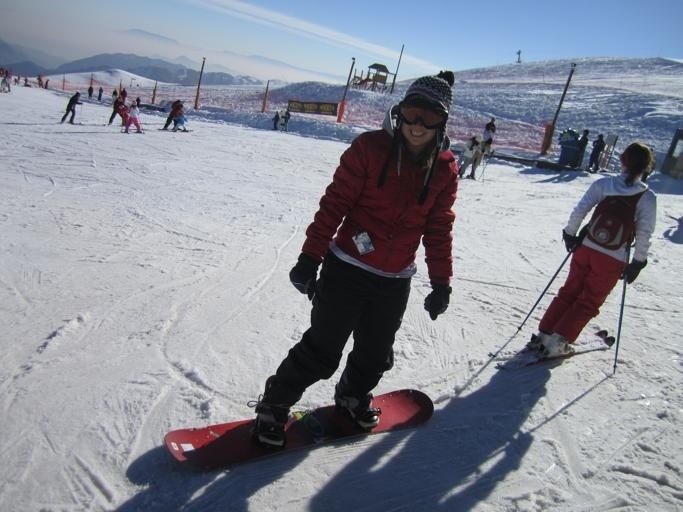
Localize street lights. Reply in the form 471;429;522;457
541;61;579;155
193;57;205;110
335;56;356;125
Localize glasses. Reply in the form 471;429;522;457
398;102;448;130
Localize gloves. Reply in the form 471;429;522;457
621;257;648;284
563;229;577;251
424;284;452;320
289;254;320;300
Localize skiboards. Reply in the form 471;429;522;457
490;330;614;369
121;130;146;135
157;128;193;132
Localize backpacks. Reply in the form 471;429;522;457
587;190;648;250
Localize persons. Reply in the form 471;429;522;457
256;70;457;450
88;86;188;133
281;109;291;131
528;143;656;360
455;117;495;179
0;68;49;92
589;133;605;171
572;130;588;168
61;92;82;124
272;111;279;129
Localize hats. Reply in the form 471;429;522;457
405;70;455;120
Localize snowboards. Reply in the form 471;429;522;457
165;390;434;471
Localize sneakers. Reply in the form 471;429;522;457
255;400;290;447
334;385;379;428
536;332;575;357
